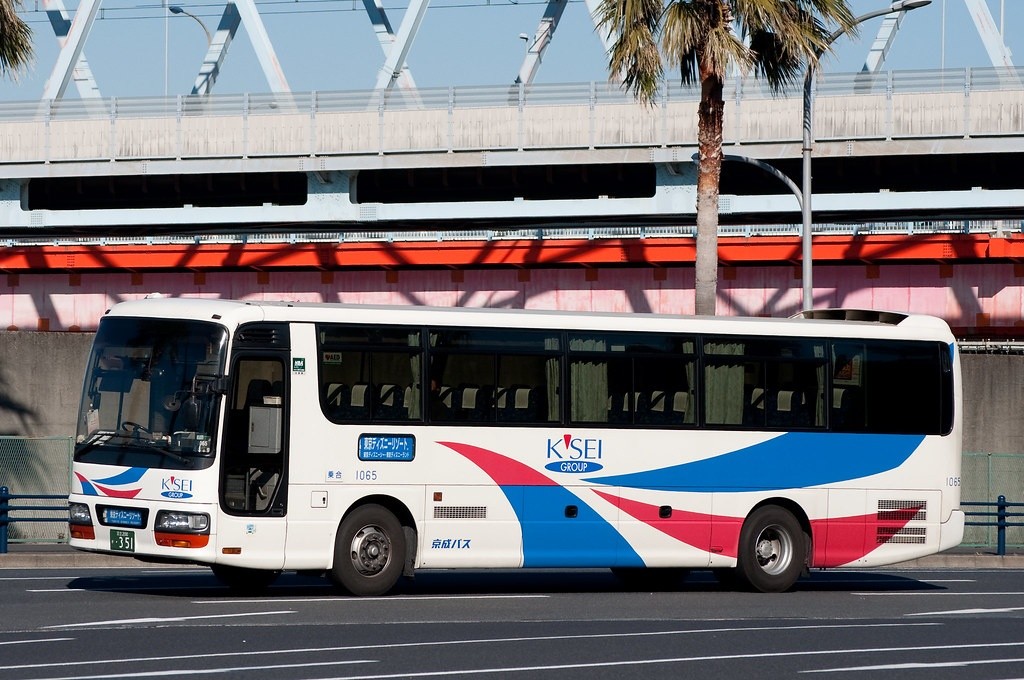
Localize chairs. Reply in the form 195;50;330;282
747;388;806;428
236;379;282;446
438;386;543;422
320;382;417;424
607;392;687;425
834;388;863;428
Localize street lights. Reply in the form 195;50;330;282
693;0;930;313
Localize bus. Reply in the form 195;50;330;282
65;298;966;595
